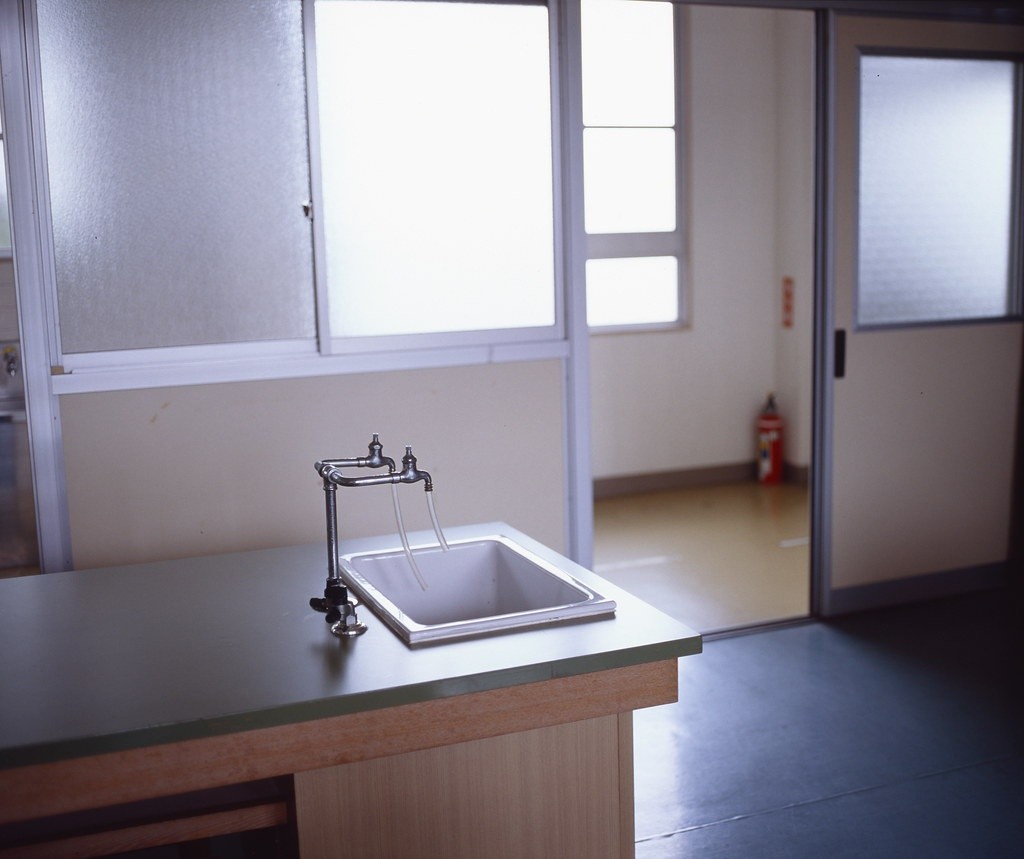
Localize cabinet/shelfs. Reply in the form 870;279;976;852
0;657;680;859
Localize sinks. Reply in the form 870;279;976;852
336;533;616;651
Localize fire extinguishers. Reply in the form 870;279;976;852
756;394;786;484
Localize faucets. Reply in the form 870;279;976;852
390;445;433;492
356;433;396;473
